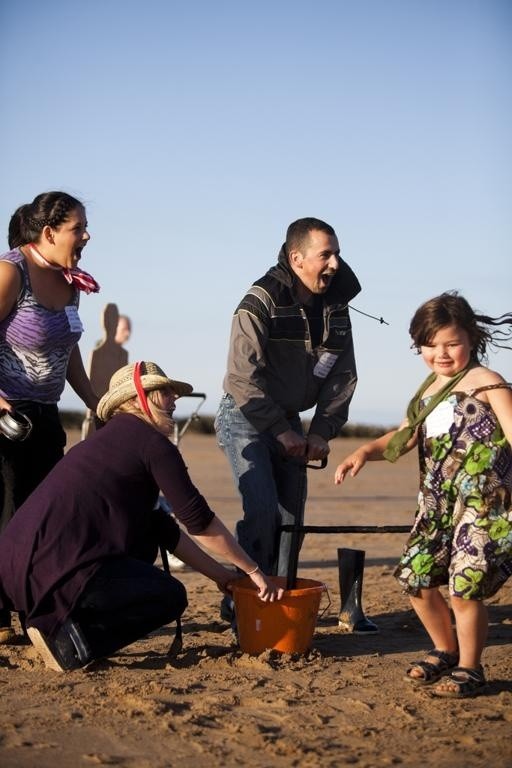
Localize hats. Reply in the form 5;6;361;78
96;361;195;422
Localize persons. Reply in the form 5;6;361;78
0;190;103;530
331;288;512;701
3;359;286;674
83;302;134;441
210;214;363;643
82;315;131;439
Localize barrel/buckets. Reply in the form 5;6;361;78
226;575;333;655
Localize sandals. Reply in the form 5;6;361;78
422;661;493;705
402;647;457;685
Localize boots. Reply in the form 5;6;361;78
333;544;385;640
219;516;291;631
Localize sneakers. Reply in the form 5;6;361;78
21;613;82;677
